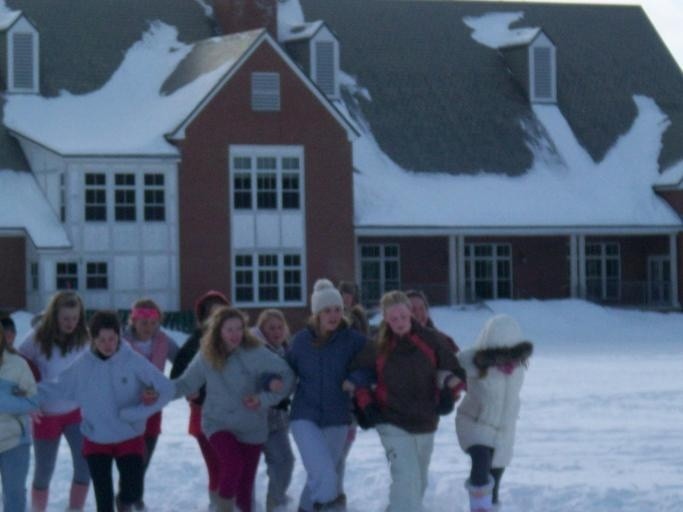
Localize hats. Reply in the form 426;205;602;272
88;309;119;339
311;279;344;313
194;291;230;315
131;300;161;320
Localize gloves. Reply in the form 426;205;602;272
446;377;467;395
242;395;261;410
138;389;160;406
358;390;381;423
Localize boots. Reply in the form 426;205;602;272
216;495;237;511
208;489;218;509
32;486;50;510
464;474;495;512
69;483;89;510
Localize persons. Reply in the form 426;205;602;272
170;290;233;511
253;309;295;511
406;289;459;360
11;309;175;512
435;315;534;512
0;324;36;512
260;278;377;512
120;299;200;511
16;288;95;512
337;279;370;483
142;305;296;512
352;289;466;512
3;319;42;396
